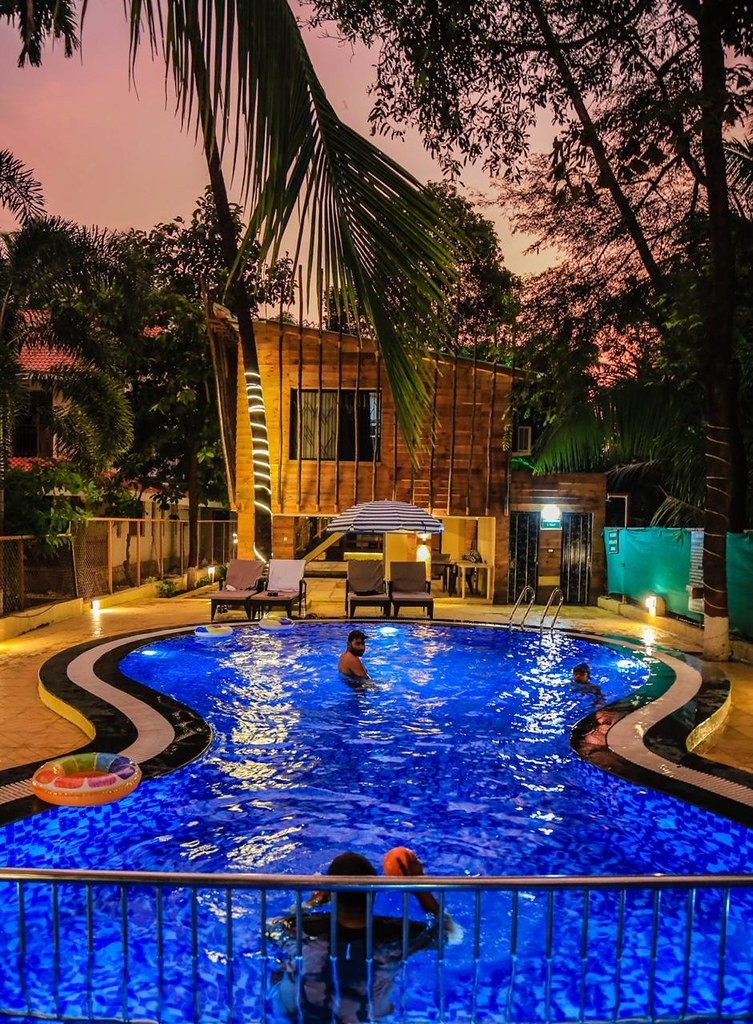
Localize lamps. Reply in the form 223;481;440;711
645;595;667;616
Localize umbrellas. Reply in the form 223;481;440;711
325;497;444;578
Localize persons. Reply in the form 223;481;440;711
338;630;370;679
572;664;591;683
294;851;443;939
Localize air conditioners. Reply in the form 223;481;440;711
517;425;532;453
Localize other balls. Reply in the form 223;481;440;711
384;844;418;880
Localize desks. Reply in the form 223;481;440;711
451;559;491;599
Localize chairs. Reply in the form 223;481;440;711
345;559;434;619
210;559;307;620
431;554;450;592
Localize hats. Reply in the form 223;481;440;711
348;631;370;642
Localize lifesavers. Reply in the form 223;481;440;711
30;751;144;811
194;622;233;639
258;616;297;632
